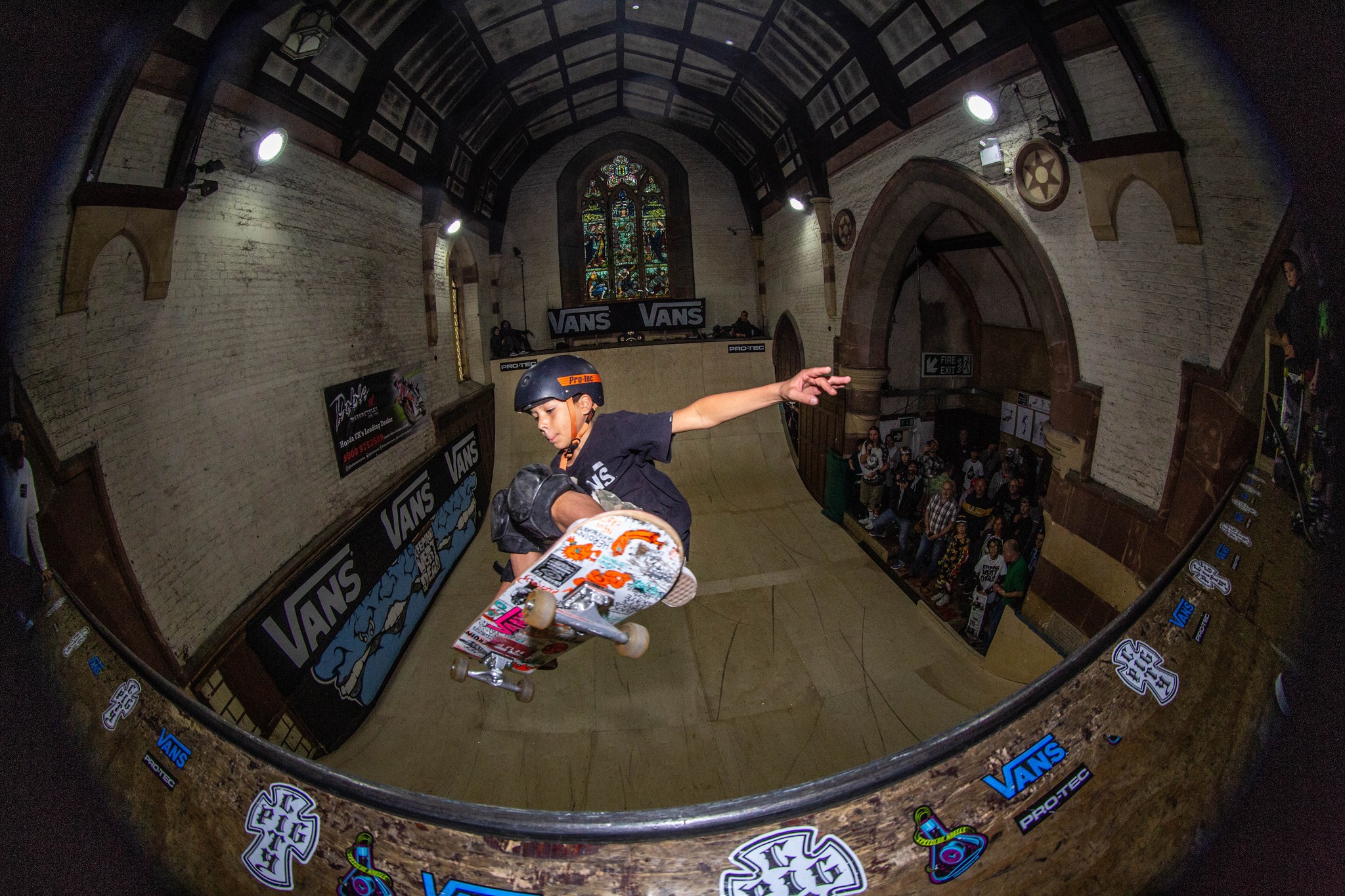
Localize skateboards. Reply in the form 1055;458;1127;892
1264;392;1321;548
448;510;685;703
963;589;988;641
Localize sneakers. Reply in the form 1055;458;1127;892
963;585;975;595
865;519;874;530
936;594;951;606
958;574;971;586
869;529;886;537
931;592;947;601
859;515;873;524
891;559;906;569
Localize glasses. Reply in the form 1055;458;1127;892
990;545;999;549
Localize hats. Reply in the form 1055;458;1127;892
955;513;968;523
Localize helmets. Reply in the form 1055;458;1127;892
514;354;605;413
899;446;912;454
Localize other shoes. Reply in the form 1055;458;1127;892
524;345;536;353
565;517;698;608
902;570;919;579
517;349;529;354
509;350;519;356
921;571;933;584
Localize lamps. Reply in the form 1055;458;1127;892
278;0;340;59
185;159;226;198
492;279;502;287
962;91;1000;125
1035;116;1073;148
439;216;462;235
787;195;809;210
246;126;287;165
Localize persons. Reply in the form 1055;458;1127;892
1274;250;1345;535
491;354;852;671
0;414;54;586
781;402;799;458
712;310;761;338
849;426;1046;648
490;320;534;356
